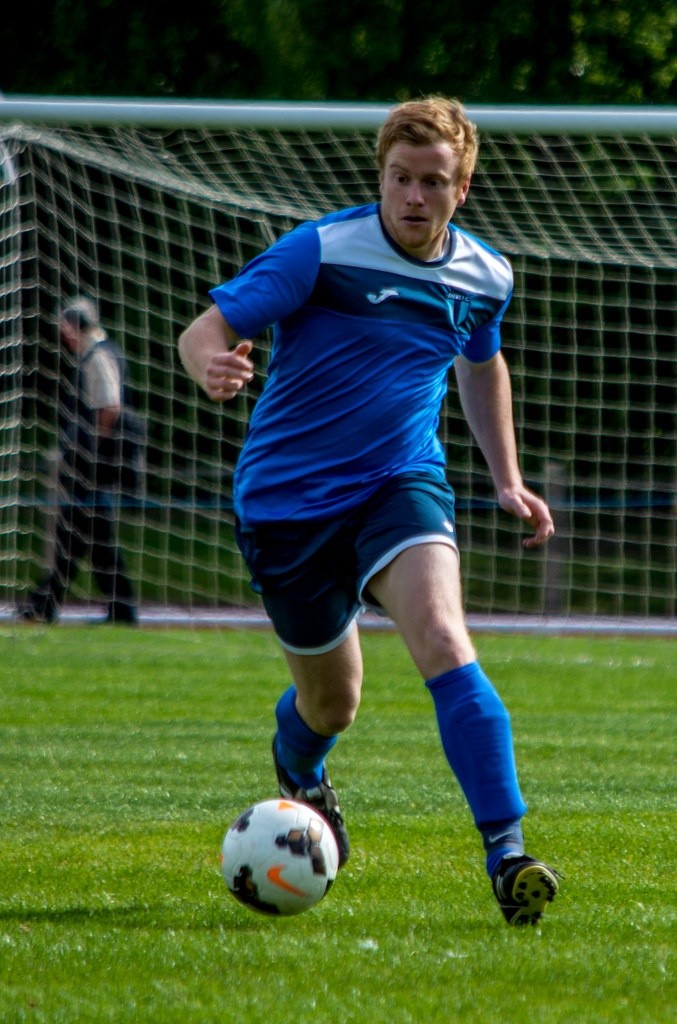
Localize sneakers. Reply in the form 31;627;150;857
491;852;566;927
271;733;350;870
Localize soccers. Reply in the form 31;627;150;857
220;799;339;918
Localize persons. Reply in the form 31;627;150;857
181;97;559;930
21;299;137;628
